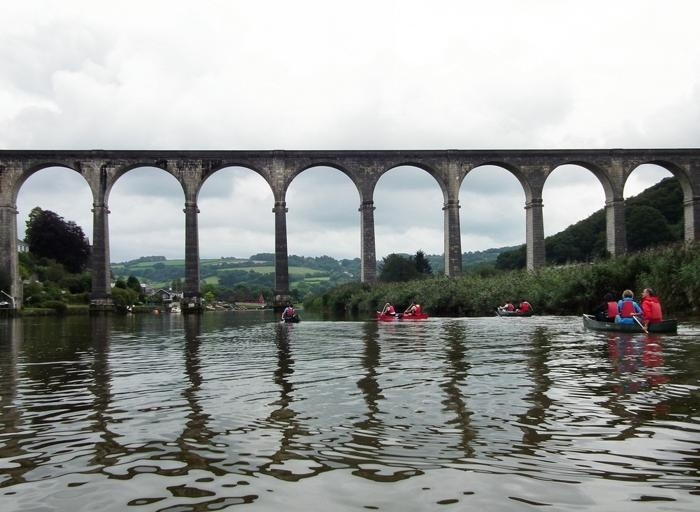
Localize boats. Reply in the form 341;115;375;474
581;311;679;336
171;307;182;313
497;305;534;317
377;310;428;322
279;313;300;323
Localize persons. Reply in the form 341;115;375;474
281;304;295;320
639;287;663;324
613;289;643;324
593;293;619;322
500;301;515;311
383;302;397;316
514;298;534;313
403;300;422;316
